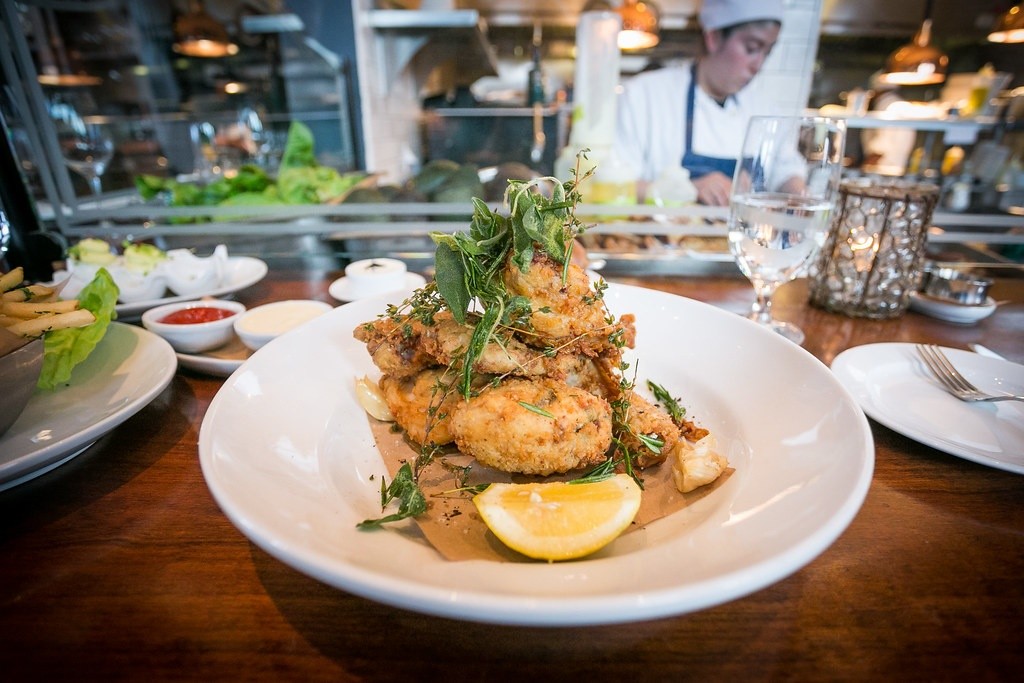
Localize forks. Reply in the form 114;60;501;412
917;342;1023;403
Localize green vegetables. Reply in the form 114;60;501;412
356;280;468;530
433;147;688;530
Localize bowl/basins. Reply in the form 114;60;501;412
0;334;43;438
917;261;993;305
52;246;406;349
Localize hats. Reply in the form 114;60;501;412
700;0;784;31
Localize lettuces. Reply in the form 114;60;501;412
134;121;367;223
36;266;122;388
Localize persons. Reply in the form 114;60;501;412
555;0;808;214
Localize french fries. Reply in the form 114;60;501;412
0;266;96;337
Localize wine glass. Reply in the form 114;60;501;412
725;114;847;346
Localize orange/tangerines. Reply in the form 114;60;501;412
472;474;641;562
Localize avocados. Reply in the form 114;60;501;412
335;159;535;222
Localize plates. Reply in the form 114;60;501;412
197;277;875;627
910;290;998;324
0;320;178;493
830;342;1023;475
38;256;428;378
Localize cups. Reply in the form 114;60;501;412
47;86;273;228
806;176;941;318
